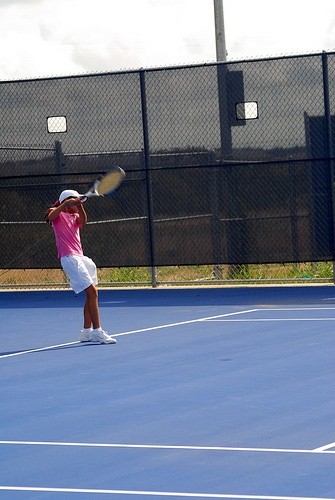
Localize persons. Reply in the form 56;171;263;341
45;190;117;344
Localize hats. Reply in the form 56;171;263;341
58;190;87;205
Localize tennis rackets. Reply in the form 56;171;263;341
77;165;126;203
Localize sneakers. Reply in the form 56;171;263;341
80;330;107;342
90;329;117;344
